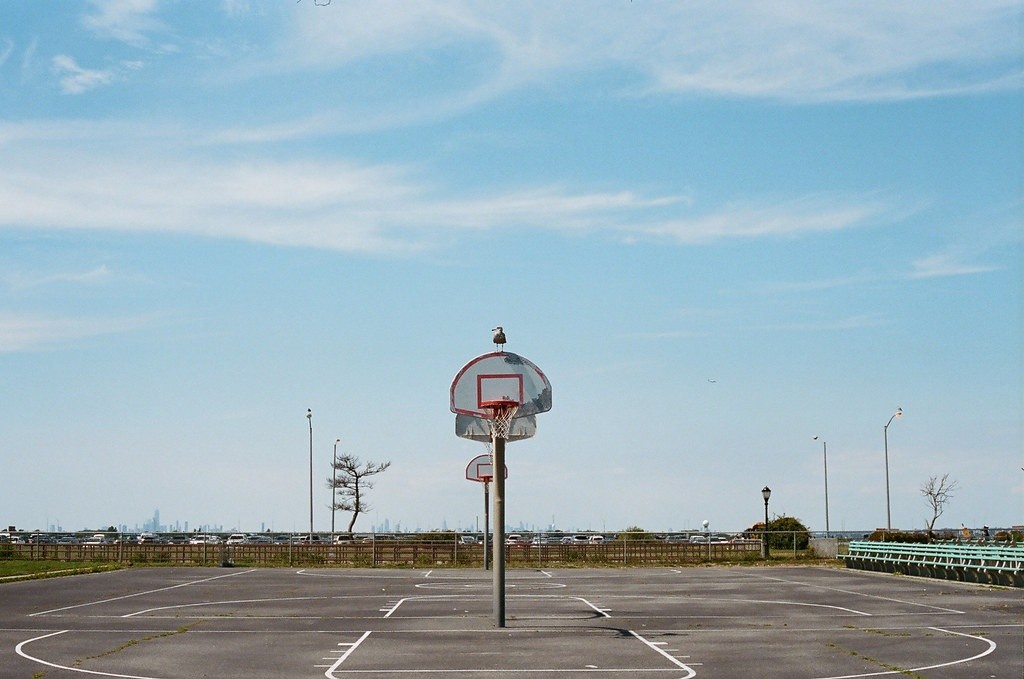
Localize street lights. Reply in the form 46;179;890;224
331;438;341;545
306;407;313;546
761;486;772;559
813;435;830;538
883;407;905;532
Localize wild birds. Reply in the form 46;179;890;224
492;327;507;352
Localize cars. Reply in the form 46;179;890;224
0;532;355;546
457;533;730;546
